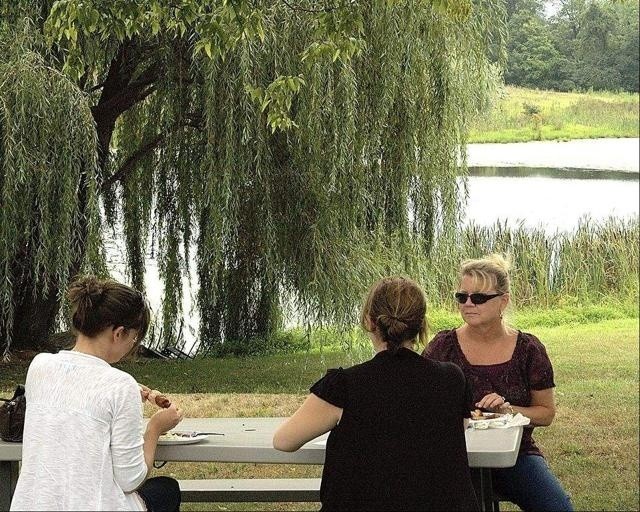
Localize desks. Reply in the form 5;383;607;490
1;416;524;511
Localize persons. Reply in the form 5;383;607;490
9;275;184;511
273;275;479;510
421;253;573;512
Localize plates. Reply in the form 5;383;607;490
156;431;202;446
469;412;508;425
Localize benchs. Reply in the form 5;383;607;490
175;478;511;511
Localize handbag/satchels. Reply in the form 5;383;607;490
0;383;28;443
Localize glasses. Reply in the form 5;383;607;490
456;292;500;305
